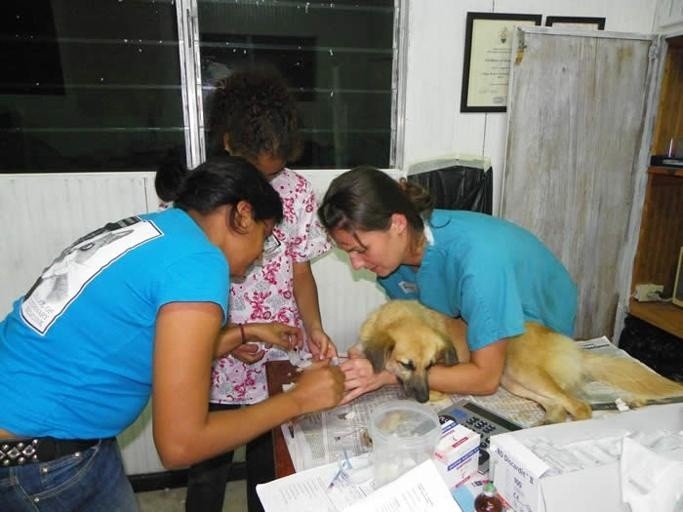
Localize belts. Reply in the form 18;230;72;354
0;433;100;468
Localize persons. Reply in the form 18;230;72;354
1;153;347;511
317;165;578;407
182;60;334;512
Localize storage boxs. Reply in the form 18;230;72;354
486;398;682;512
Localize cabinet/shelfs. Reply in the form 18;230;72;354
495;24;683;347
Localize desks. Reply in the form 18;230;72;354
264;355;325;478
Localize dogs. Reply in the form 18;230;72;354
358;298;683;428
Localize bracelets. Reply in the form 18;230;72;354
235;322;250;343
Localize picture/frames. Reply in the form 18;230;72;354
544;16;606;31
461;10;541;116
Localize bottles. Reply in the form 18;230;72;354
472;484;502;512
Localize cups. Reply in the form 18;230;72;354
658;135;682;160
370;401;441;489
